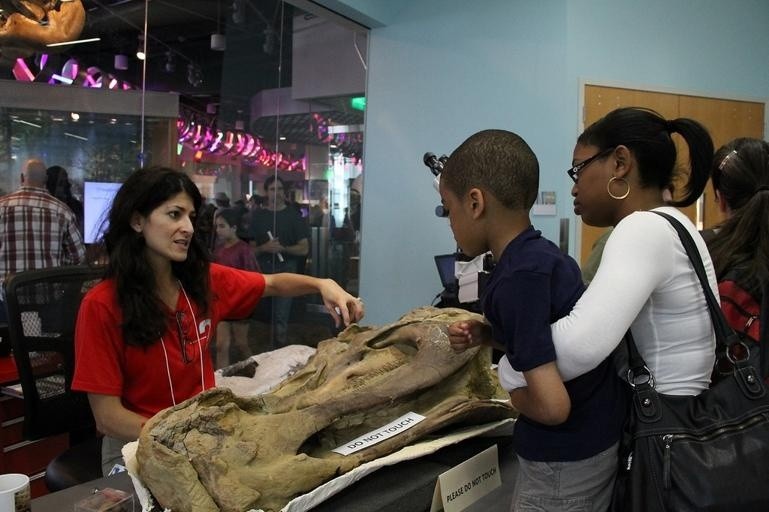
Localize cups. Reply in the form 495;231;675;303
0;473;31;512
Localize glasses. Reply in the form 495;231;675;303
567;146;612;183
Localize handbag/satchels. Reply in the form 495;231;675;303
610;342;768;511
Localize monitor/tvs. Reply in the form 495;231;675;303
79;178;124;246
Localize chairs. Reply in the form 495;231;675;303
3;264;115;494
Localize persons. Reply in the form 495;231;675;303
0;160;87;331
582;179;675;288
698;136;768;361
439;129;625;512
496;107;721;512
71;164;365;476
197;175;336;369
